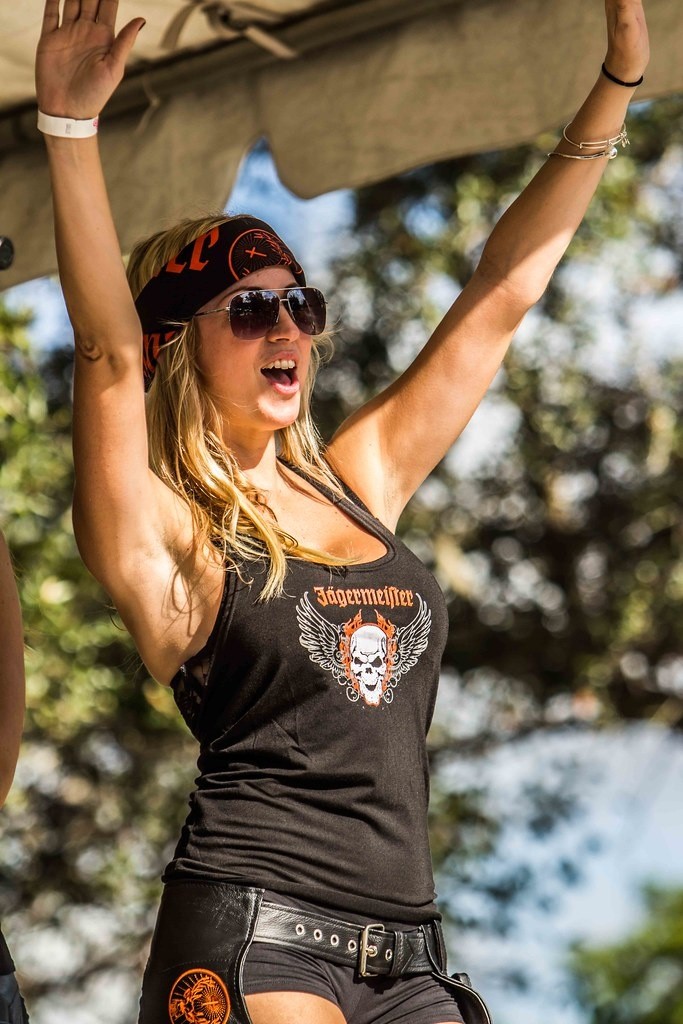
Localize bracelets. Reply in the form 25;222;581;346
599;62;644;87
547;121;630;161
35;108;100;138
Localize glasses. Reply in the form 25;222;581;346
192;286;328;341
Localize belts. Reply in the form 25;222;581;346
251;899;440;977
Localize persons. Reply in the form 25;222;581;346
36;0;650;1024
0;536;30;1024
